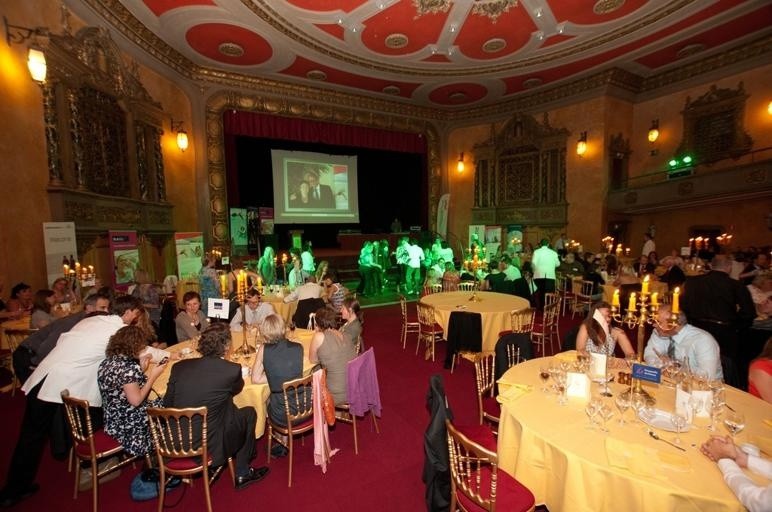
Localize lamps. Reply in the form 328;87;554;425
647;120;660;145
172;121;188;154
576;131;587;154
457;151;465;172
4;17;47;85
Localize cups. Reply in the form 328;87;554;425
740;442;760;458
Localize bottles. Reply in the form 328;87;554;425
255;326;262;353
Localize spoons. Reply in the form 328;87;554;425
649;430;685;451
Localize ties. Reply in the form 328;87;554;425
668;336;675;359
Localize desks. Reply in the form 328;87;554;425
499;351;771;511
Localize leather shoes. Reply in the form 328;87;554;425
234;465;269;489
0;484;37;507
263;444;288;457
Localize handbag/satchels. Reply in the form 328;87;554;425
130;467;186;508
320;368;335;427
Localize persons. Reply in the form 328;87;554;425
289;182;319;210
584;234;772;335
257;241;347;328
576;301;635;359
643;305;723;382
290;171;337;209
747;336;772;406
357;233;583;309
700;435;772;512
0;270;178;349
2;291;365;508
116;255;132;283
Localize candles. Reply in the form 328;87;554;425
608;272;682;327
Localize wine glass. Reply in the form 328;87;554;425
539;356;745;439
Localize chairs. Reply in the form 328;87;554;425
266;366;331;488
145;406;237;512
397;247;721;364
497;333;534;371
1;248;380;456
60;389;152;512
430;375;536;512
474;350;502;426
